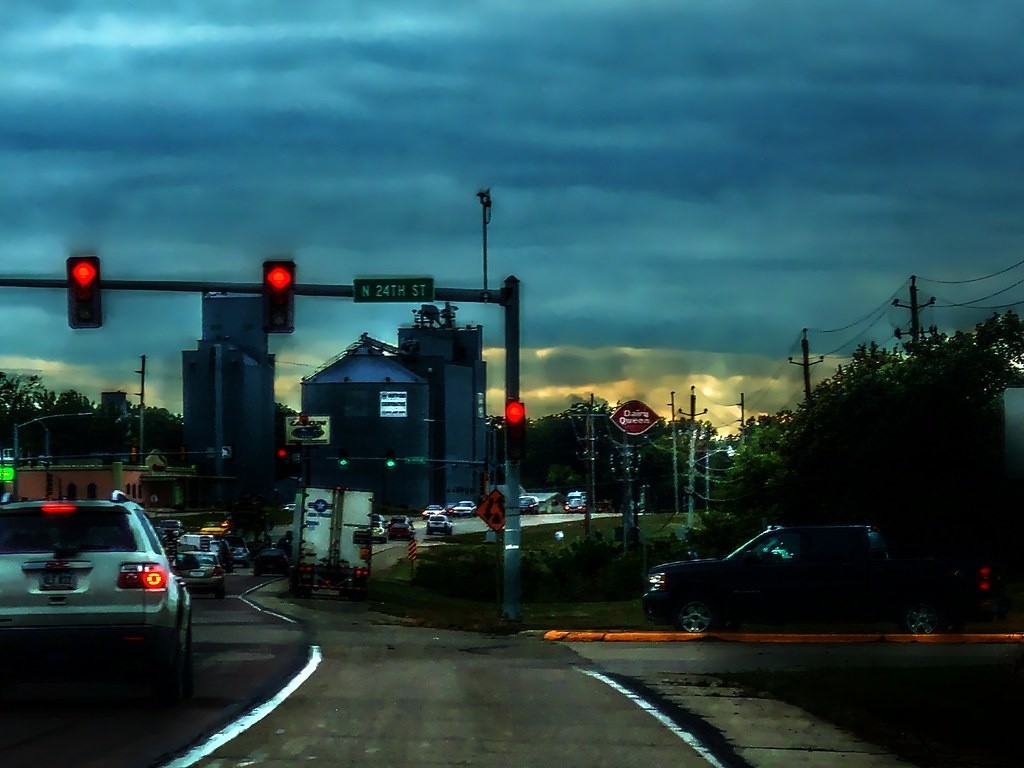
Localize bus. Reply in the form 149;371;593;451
566;492;586;504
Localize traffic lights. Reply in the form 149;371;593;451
506;402;527;447
262;259;295;333
278;446;286;462
67;257;101;328
340;449;348;468
386;451;395;468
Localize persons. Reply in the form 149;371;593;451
264;534;272;546
178;555;197;569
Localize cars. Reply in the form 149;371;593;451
373;514;384;523
422;505;446;519
520;500;539;514
156;519;183;538
254;549;290;576
200;521;228;537
373;521;388;543
172;551;225;599
389;523;411;540
641;523;989;638
389;516;408;530
565;498;586;513
445;503;459;514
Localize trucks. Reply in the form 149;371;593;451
291;487;373;595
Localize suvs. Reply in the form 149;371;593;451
275;538;292;554
427;514;452;536
0;490;193;706
203;540;234;572
223;536;250;568
449;501;477;517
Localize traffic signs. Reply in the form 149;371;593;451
353;278;434;303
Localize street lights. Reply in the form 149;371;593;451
13;412;92;466
